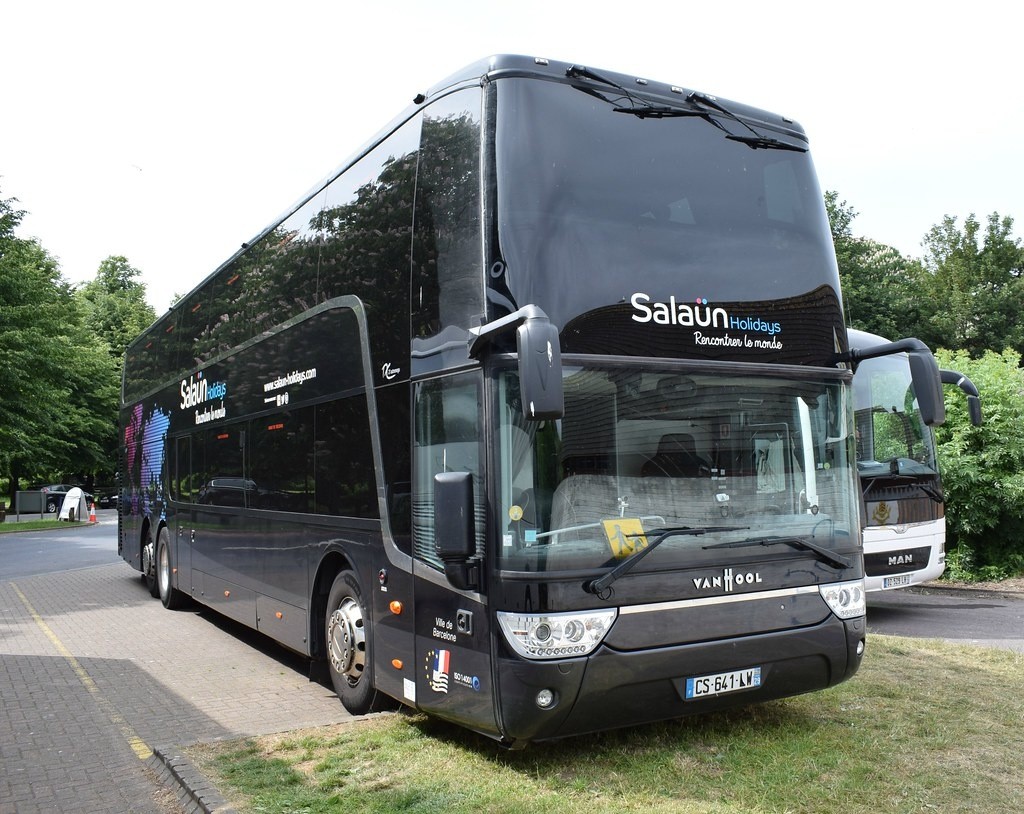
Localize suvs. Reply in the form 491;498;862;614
199;475;292;511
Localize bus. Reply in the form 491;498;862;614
116;54;947;749
760;327;982;593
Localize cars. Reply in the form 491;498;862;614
98;489;118;508
384;481;429;513
26;484;95;513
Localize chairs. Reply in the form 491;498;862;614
640;434;714;494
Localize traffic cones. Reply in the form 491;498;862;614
88;502;97;523
